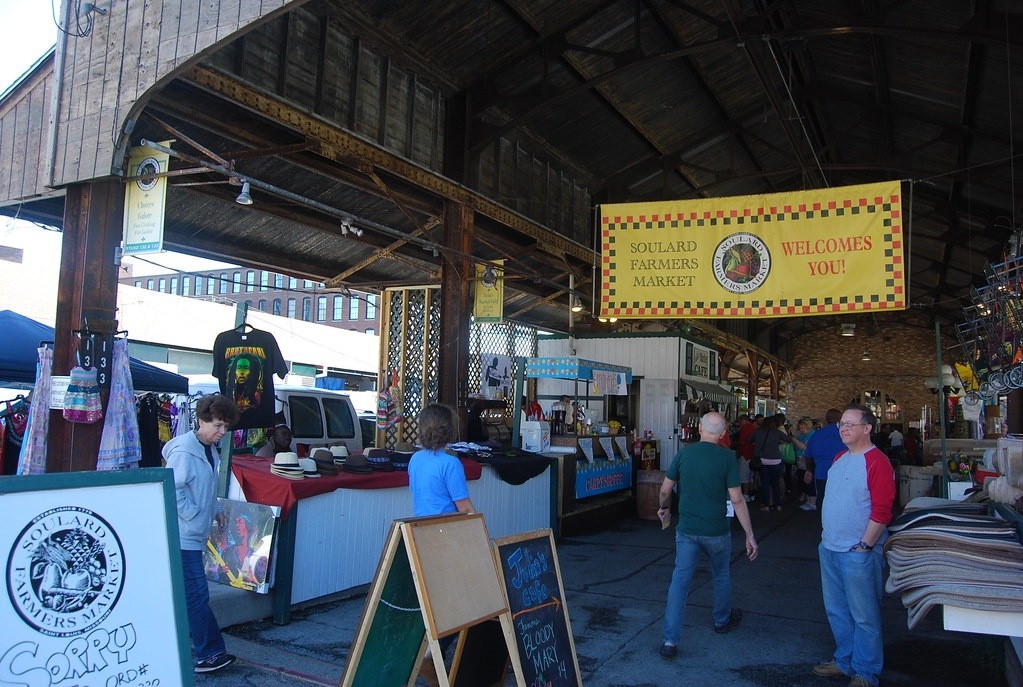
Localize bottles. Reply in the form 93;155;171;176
532;410;592;436
681;417;700;442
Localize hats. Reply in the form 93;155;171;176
267;441;416;481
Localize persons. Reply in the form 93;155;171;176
560;395;570;405
718;408;849;511
521;394;545;421
870;423;920;465
255;425;293;458
161;396;236;673
657;412;760;656
408;403;477;517
810;404;896;687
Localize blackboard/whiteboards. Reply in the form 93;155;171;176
338;511;511;687
447;528;583;687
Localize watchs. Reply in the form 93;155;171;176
859;540;872;550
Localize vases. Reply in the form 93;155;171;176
610;428;619;436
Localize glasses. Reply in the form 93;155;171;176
836;421;867;429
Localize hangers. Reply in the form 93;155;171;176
234;314;255;332
114;328;128;342
0;385;31;406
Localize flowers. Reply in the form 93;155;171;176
609;420;620;428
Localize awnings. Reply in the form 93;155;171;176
680;377;738;404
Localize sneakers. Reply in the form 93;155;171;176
195;653;235;673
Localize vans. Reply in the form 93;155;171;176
159;382;364;458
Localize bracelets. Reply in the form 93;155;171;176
660;506;668;510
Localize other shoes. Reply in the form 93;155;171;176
811;662;841;676
777;505;783;511
849;676;870;687
661;641;677;657
799;496;805;501
760;506;770;512
715;608;742;633
800;503;817;510
744;495;755;502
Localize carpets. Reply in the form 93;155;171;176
880;498;1023;630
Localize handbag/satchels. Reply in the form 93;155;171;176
738;456;752;480
749;456;762;471
781;442;795;464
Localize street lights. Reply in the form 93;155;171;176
924;364;962;439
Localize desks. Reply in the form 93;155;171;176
230;446;558;627
938;604;1023;638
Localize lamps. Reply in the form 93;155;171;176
236;181;253;205
572;296;582;312
841;327;855;335
348;223;363;237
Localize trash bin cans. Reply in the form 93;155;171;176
636;470;667;523
